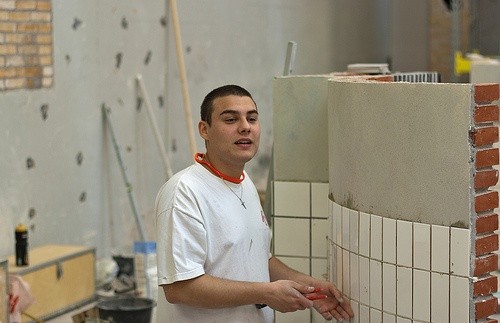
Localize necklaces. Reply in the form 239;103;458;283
194;152;246;182
221;177;247;209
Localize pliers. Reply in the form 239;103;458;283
299;288;328;302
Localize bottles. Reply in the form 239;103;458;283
15;223;28;266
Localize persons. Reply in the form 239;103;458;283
150;85;354;323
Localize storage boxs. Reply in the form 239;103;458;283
7;245;98;323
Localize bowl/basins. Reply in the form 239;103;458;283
96;298;157;323
